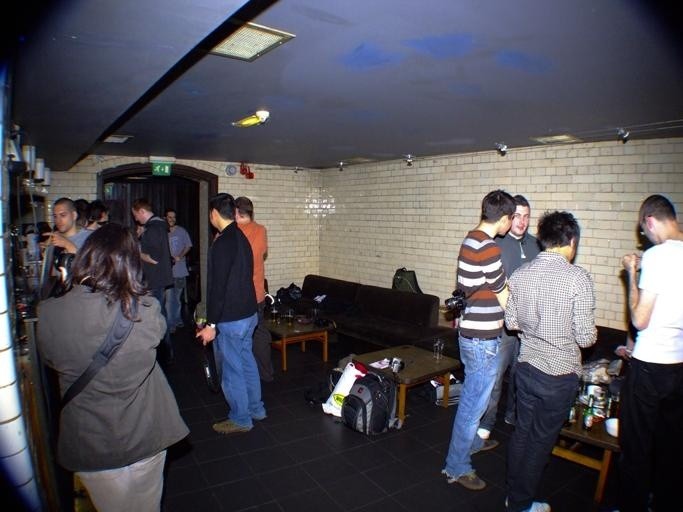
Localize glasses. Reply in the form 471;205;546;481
638;214;652;236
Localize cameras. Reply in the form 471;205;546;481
54;254;74;271
445;289;467;311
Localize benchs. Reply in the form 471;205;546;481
297;272;438;348
579;325;630;369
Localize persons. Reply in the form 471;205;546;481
614;194;683;512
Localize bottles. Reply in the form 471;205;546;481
16;239;30;266
566;371;619;431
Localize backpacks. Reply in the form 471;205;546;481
390;266;423;298
340;369;400;437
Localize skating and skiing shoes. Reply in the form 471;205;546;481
480;438;500;452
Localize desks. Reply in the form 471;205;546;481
265;314;331;371
351;343;461;422
549;376;628;506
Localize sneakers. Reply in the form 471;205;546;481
212;418;252;436
443;465;486;491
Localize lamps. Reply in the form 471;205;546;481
403;153;413;166
294;166;299;174
615;127;630;144
495;141;507;156
337;160;345;171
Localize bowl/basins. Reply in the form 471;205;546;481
605;418;619;438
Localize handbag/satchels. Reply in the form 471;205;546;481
321;361;367;418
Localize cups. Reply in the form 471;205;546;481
270;305;321;326
433;337;445;361
